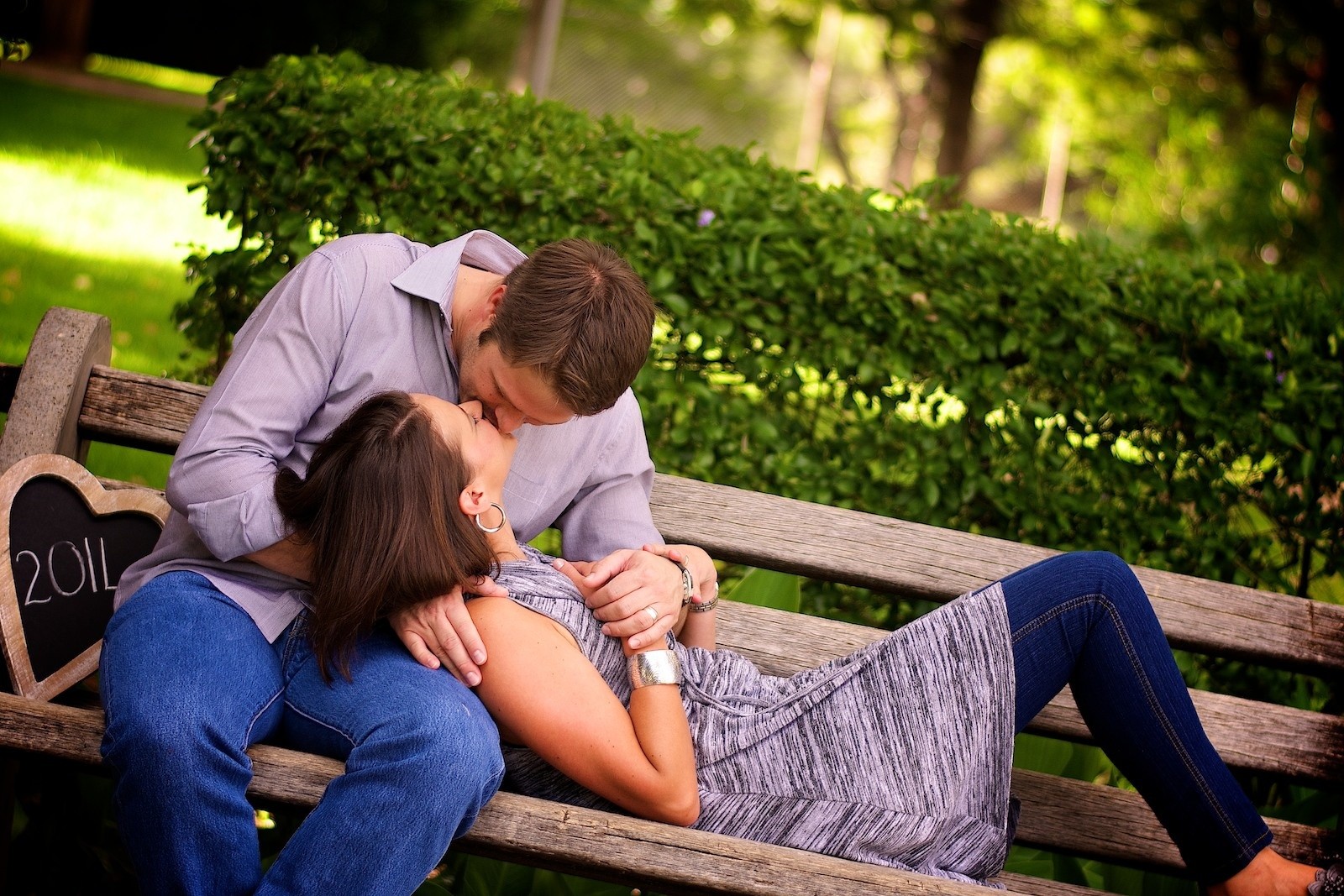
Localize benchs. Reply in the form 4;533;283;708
0;304;1343;893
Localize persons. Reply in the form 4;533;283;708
105;229;696;896
302;391;1344;896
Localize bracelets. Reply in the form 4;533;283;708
689;583;719;612
625;648;682;690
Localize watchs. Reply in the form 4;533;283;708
662;554;693;607
